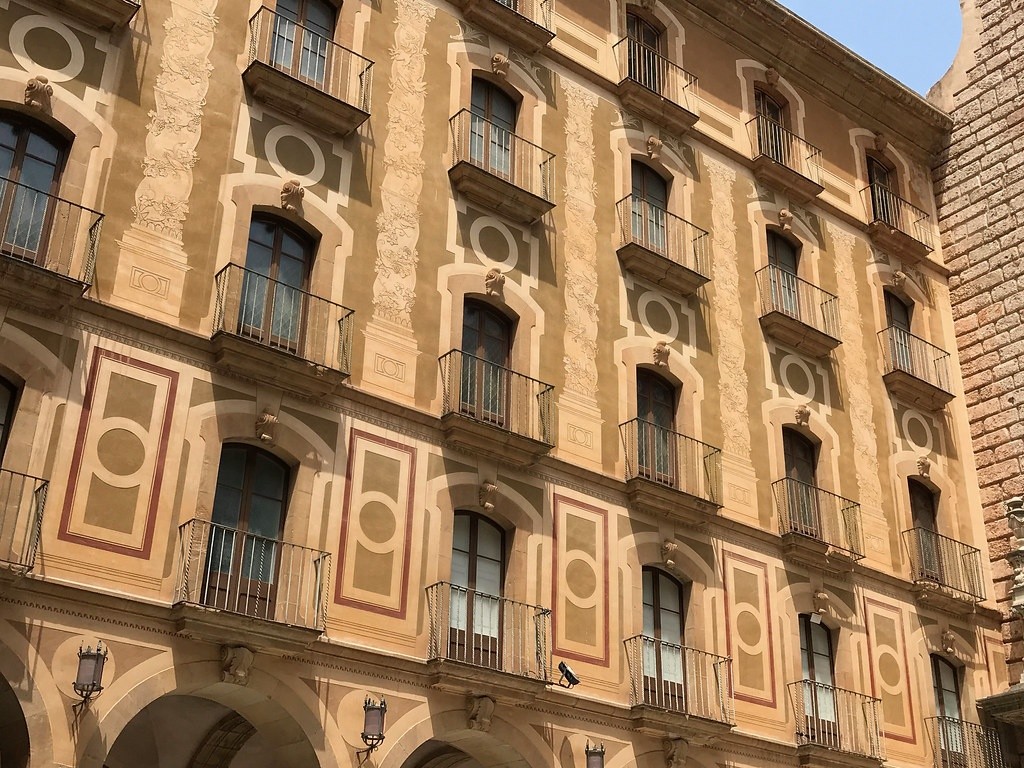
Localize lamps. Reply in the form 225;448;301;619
585;739;605;768
550;660;582;689
356;694;387;768
71;639;108;726
